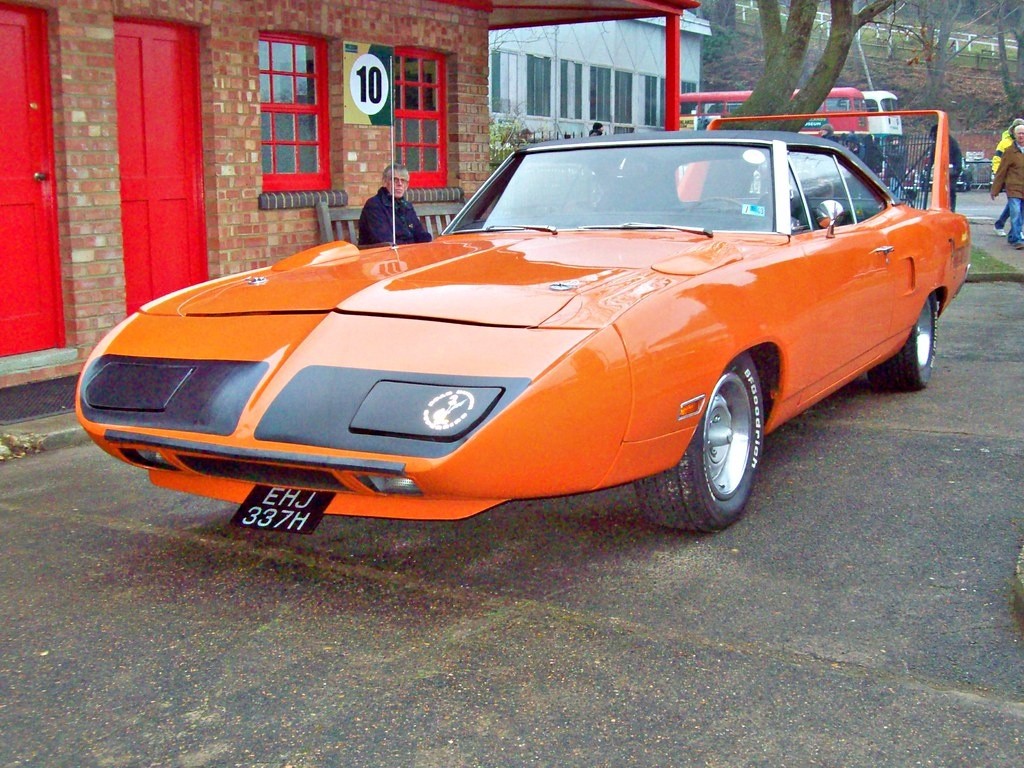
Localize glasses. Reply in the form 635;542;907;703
390;176;406;184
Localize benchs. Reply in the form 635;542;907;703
317;196;470;244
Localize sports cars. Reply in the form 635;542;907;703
75;110;971;536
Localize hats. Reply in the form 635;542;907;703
1012;118;1024;126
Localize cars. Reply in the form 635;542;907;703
917;156;972;192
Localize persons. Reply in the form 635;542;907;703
358;163;432;245
927;124;962;213
817;124;907;200
589;123;604;136
990;119;1024;249
518;128;532;149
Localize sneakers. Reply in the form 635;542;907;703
1020;232;1024;239
993;226;1007;237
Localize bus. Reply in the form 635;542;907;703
678;87;871;173
690;91;905;174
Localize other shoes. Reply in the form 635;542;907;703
1013;243;1024;249
